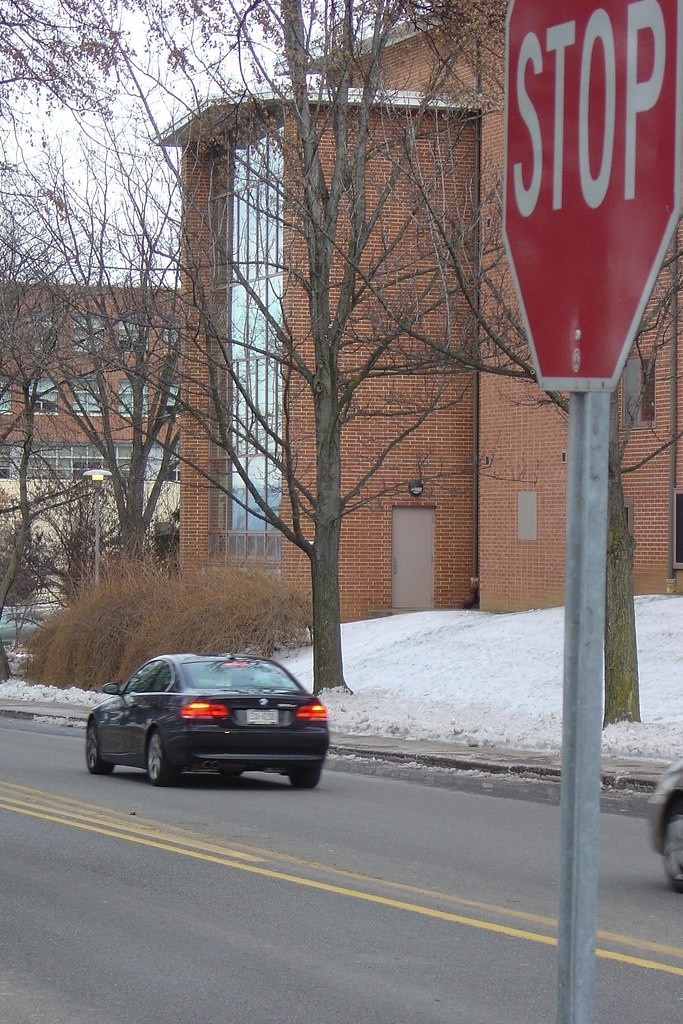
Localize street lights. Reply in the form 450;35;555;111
81;462;114;587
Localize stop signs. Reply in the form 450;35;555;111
499;0;682;389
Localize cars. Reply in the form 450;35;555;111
648;757;683;893
84;653;332;787
